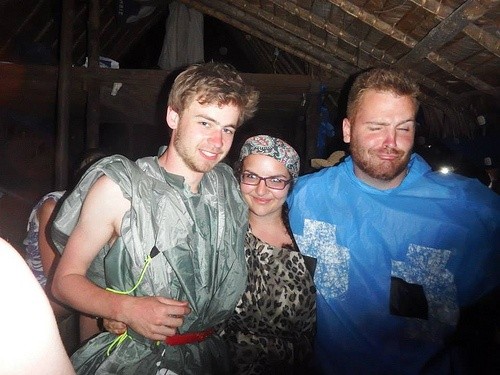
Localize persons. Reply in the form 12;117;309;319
103;129;317;375
286;68;500;375
51;62;260;375
0;151;349;375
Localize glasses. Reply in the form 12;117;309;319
238;172;292;190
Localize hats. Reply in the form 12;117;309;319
239;135;300;184
